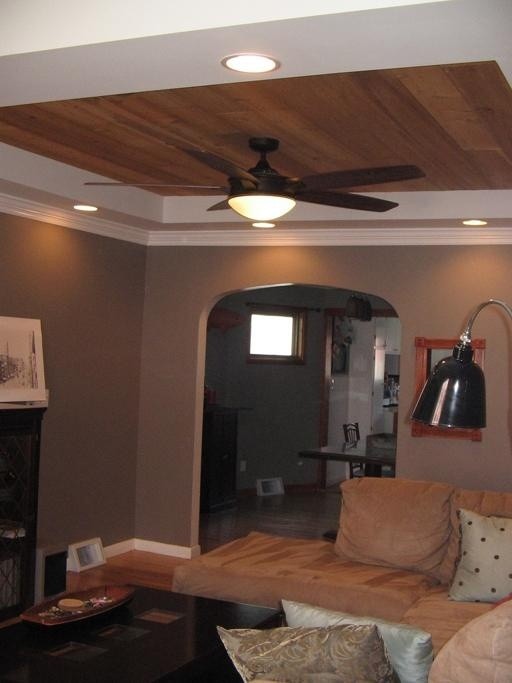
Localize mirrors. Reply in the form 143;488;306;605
409;332;486;443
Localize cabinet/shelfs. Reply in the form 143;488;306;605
0;406;48;624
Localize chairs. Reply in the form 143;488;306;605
340;424;367;477
365;432;396;479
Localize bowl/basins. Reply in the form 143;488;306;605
22;578;138;627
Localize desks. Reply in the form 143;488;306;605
302;439;396;538
2;587;281;681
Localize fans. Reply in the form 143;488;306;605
74;128;424;214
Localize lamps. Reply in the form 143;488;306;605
225;190;297;220
411;298;509;435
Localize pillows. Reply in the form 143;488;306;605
336;473;451;576
218;620;390;680
449;507;512;600
279;600;432;678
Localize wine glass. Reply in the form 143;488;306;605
386;377;396;404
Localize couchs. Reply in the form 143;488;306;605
177;528;512;681
